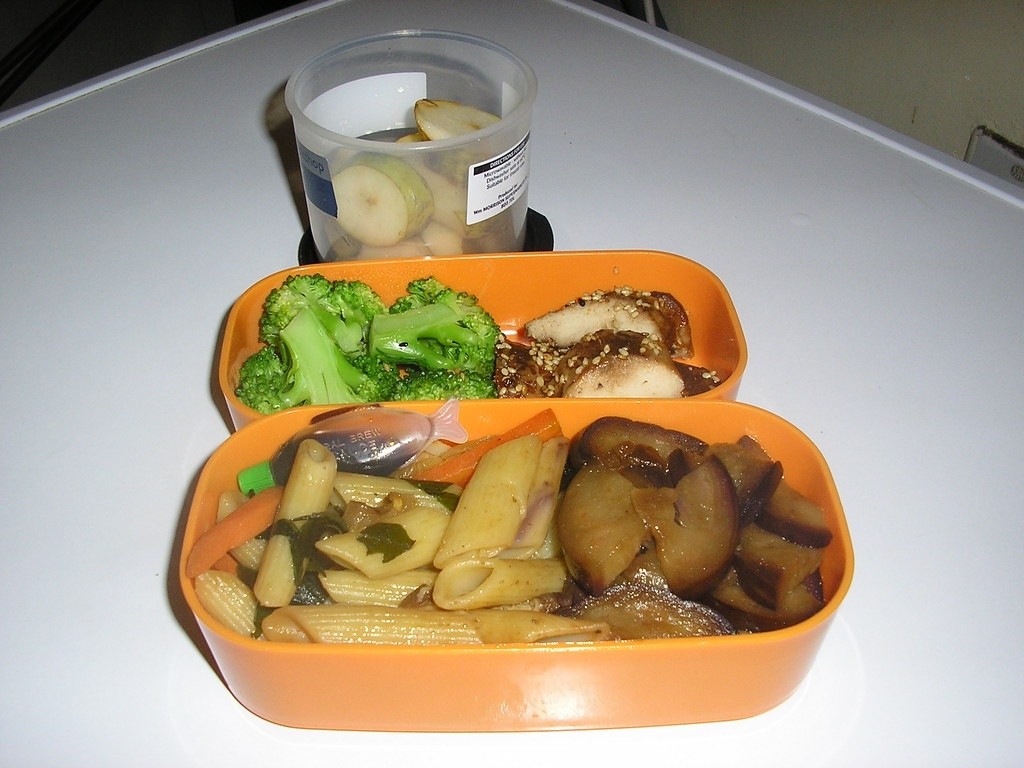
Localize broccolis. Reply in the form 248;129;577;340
236;272;500;419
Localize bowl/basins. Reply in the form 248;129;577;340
177;397;855;733
220;247;748;405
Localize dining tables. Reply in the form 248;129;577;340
0;0;1024;768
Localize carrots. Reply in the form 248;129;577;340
399;406;565;488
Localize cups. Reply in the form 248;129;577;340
283;25;537;263
